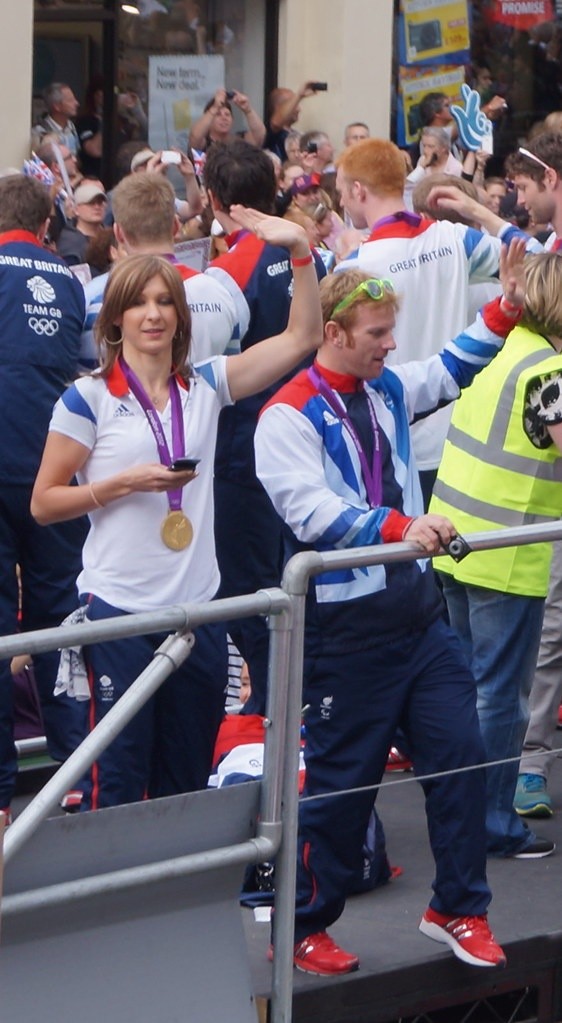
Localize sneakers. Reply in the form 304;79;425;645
384;746;415;772
417;906;507;967
513;836;557;858
513;774;554;818
267;929;361;978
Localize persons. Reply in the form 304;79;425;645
422;251;562;862
511;526;562;819
28;203;326;810
1;175;91;832
335;139;513;775
28;1;562;325
256;233;531;980
201;139;338;729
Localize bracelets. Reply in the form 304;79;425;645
88;481;106;508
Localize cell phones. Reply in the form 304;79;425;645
161;150;182;164
168;458;201;471
312;83;327;90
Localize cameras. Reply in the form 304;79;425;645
224;90;235;99
436;527;472;563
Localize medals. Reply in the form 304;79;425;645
160;511;194;553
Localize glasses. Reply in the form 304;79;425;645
512;143;550;170
330;278;396;320
63;151;74;161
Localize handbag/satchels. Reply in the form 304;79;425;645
207;712;392;895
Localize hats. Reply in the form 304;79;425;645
74;184;109;203
131;150;156;172
291;173;322;194
